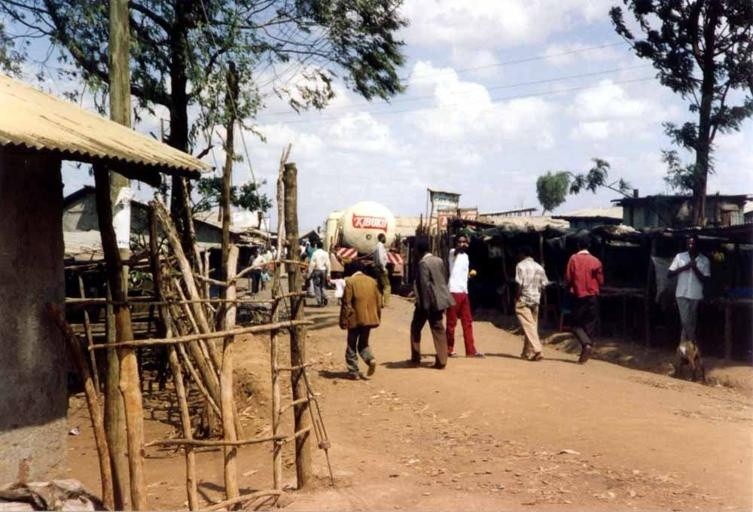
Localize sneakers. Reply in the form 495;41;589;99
368;358;376;376
345;373;361;380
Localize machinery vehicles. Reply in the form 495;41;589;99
325;201;404;294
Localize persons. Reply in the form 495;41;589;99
271;244;277;261
567;238;604;364
330;273;346;304
668;233;711;342
263;247;273;274
402;238;454;370
250;249;263;295
373;233;394;308
307;242;331;307
513;244;548;361
260;266;269;291
444;231;483;358
339;262;384;381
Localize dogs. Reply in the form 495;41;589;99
668;339;705;382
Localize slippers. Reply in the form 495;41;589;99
448;351;458;358
468;351;484;358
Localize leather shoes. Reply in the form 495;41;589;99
532;352;544;360
580;345;592;363
521;352;531;360
